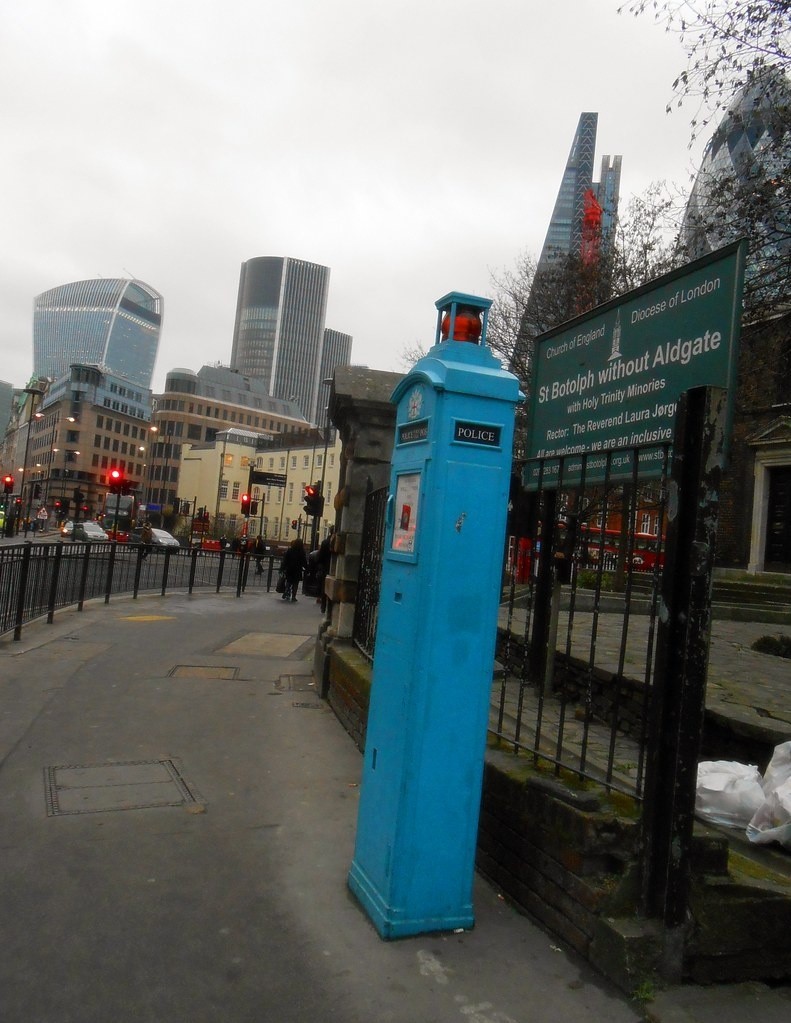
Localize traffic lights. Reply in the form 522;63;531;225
122;480;130;495
174;497;181;514
252;502;258;515
240;494;249;514
197;507;203;520
204;512;209;521
4;476;13;494
84;504;88;512
183;503;189;514
73;488;79;502
303;485;320;515
107;469;122;494
16;499;21;508
292;520;297;529
55;502;60;510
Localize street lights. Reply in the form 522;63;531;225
151;426;170;518
35;412;75;506
53;449;80;507
310;377;334;554
16;387;45;536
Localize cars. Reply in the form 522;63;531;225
62;521;74;536
71;523;108;542
131;527;180;550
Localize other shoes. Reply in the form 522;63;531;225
282;592;291;600
321;608;327;614
291;597;298;602
255;569;263;575
140;555;148;562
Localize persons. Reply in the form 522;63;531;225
278;538;310;603
319;525;334;613
141;520;153;561
254;535;266;575
220;534;226;549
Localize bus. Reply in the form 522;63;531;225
557;523;664;571
100;494;134;542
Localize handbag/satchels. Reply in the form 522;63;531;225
275;576;287;594
